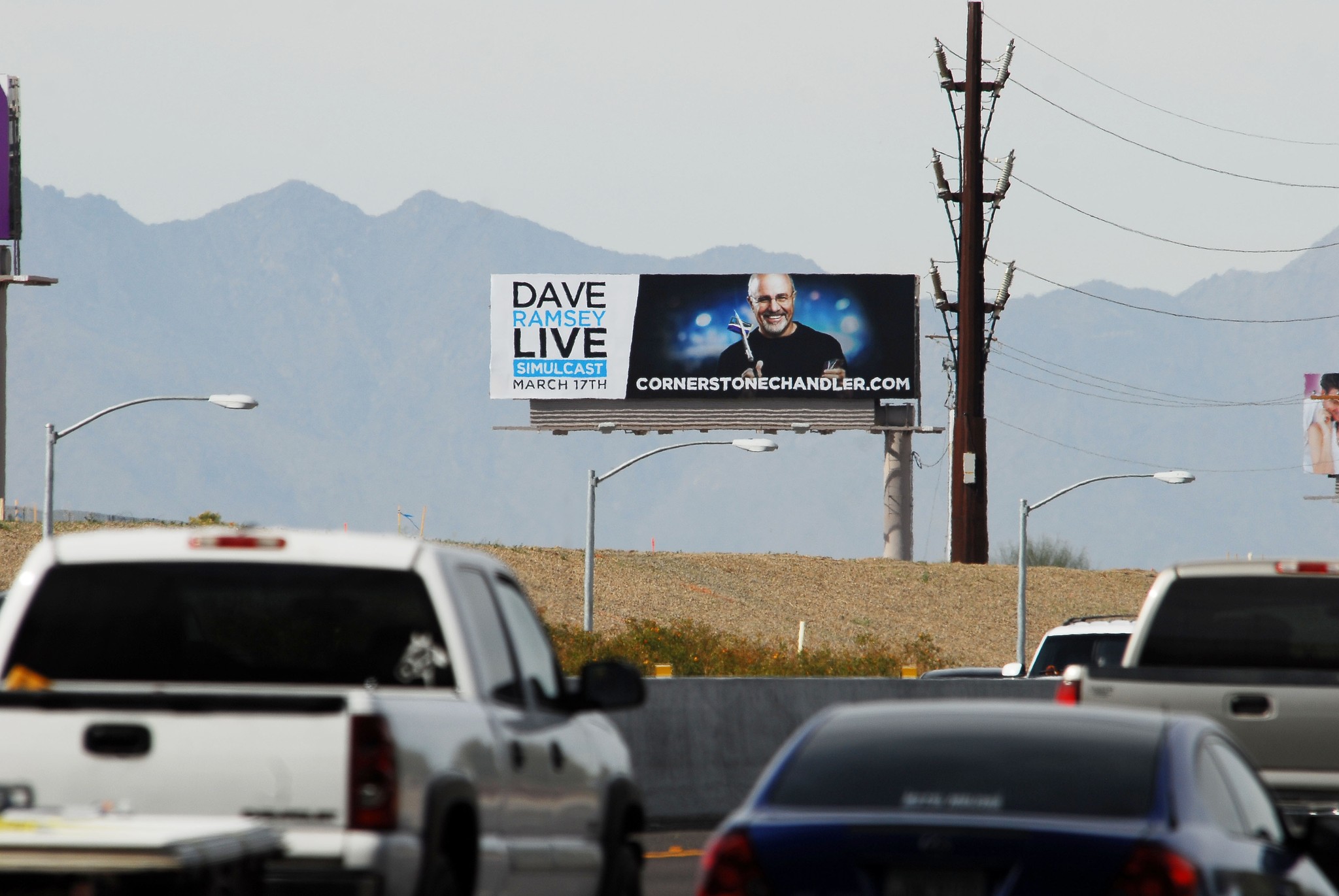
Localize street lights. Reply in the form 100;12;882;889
44;393;259;535
584;437;778;633
1015;470;1195;668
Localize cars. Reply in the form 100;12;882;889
697;701;1339;896
918;668;1002;679
0;810;301;896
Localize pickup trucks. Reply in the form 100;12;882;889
0;527;650;896
1058;560;1339;837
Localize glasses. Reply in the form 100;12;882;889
750;291;794;305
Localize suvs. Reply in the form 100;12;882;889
1000;615;1136;680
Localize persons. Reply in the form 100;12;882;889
719;274;849;396
1305;373;1339;474
